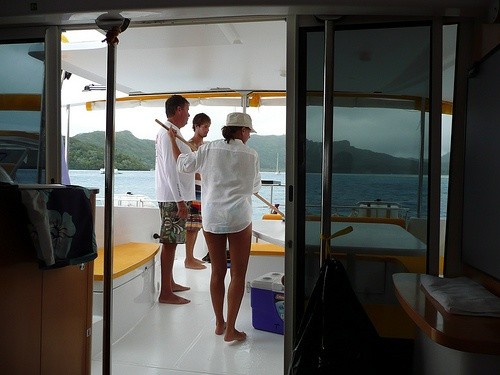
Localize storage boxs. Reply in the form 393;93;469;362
249;269;286;336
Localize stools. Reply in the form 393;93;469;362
245;243;285;294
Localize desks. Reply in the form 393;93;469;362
304;215;500;375
252;219;285;248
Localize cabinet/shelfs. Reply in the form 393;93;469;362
0;181;100;374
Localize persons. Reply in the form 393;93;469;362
155;95;196;304
185;113;211;269
168;113;263;342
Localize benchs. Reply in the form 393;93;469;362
94;241;160;328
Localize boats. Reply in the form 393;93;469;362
100;167;119;175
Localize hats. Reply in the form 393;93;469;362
226;112;257;133
